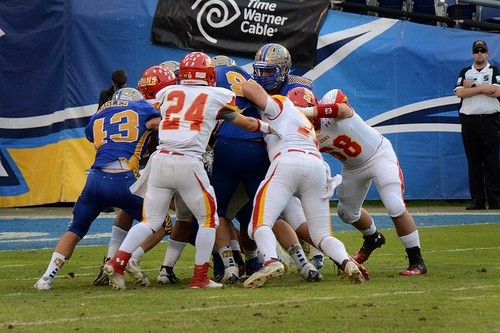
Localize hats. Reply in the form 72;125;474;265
112;70;127;85
473;40;488;51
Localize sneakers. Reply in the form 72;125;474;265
190;275;223;288
103;258;126;290
93;257;112;285
157;268;180;285
352;231;385;264
34;276;53;290
124;258;150;286
212;239;369;288
400;263;427;275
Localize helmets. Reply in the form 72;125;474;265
112;88;143;101
159;61;180;85
210;55;237;67
252;44;292;92
138;66;177;100
179;52;216;87
286;87;319;129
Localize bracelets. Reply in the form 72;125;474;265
471;83;476;88
253;118;272;134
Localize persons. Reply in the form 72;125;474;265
98;43;369;284
244;79;365;287
453;40;500;210
103;52;282;290
35;88;172;291
286;88;428;277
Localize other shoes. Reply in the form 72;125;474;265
489;205;500;209
466;203;485;210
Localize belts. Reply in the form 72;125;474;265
273;150;319;159
160;150;184;156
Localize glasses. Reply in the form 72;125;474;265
474;48;486;53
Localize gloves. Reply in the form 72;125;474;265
263;127;283;140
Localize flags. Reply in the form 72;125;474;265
151;0;330;69
0;0;500;207
